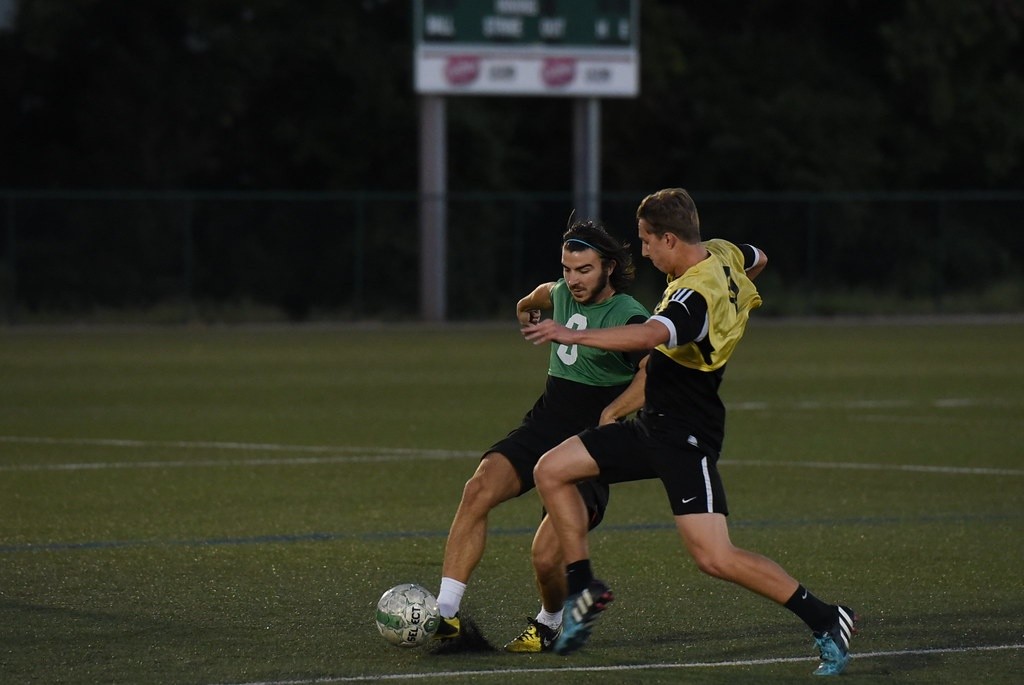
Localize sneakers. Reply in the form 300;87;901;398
814;605;858;675
431;612;461;640
554;580;615;655
504;617;563;653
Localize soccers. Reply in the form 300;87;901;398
375;583;440;648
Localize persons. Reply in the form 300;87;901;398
421;220;651;654
518;188;860;679
